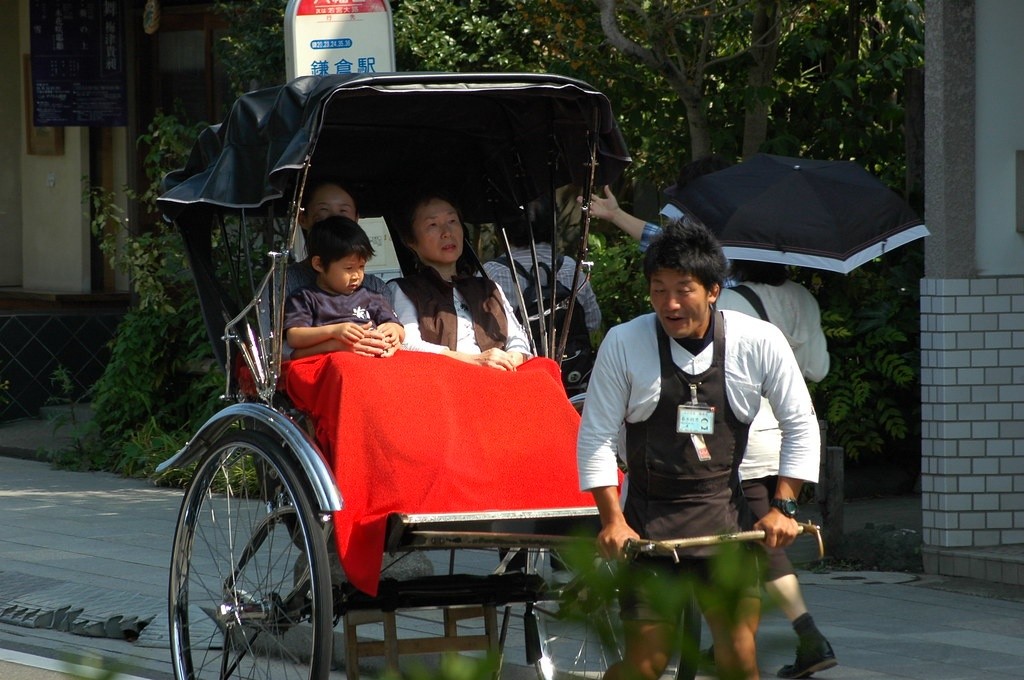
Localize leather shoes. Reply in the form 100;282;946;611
708;645;715;663
777;639;838;678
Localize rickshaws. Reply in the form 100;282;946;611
154;69;825;680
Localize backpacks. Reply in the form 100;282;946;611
490;253;596;397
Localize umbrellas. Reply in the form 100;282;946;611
658;152;930;274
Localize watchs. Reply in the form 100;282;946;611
770;498;800;518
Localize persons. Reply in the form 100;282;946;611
475;194;601;589
580;157;736;289
701;418;708;430
698;260;838;679
386;192;533;371
282;175;402;357
283;216;405;346
575;222;821;680
